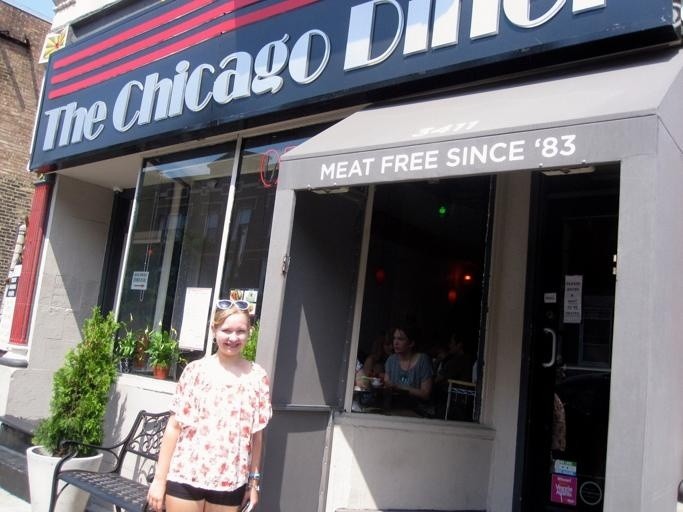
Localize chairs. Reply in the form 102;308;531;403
443;360;479;421
49;409;176;512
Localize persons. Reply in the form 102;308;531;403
146;300;271;512
354;321;474;417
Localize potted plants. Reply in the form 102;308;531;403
115;312;189;380
25;304;127;512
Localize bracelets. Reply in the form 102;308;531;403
247;484;261;493
248;472;263;481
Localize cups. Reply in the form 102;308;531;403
371;377;382;385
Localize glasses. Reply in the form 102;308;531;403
214;299;251;311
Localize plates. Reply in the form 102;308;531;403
370;383;383;388
